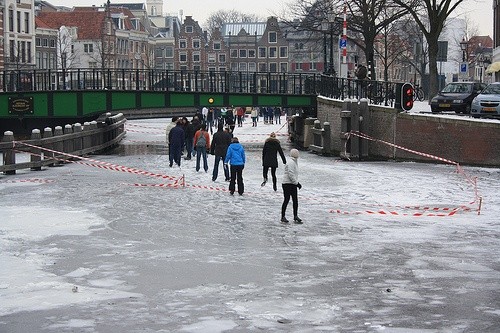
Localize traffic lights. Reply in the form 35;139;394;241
401;83;414;112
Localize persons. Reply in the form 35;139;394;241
167;107;287;197
280;149;304;224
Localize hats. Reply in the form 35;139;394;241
270;131;276;138
290;149;299;157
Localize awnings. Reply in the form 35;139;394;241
486;62;500;73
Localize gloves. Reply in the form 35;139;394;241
223;164;228;170
297;183;301;190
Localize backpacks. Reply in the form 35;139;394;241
196;134;206;147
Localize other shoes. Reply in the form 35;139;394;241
273;185;277;191
239;192;242;196
184;157;190;160
294;216;301;223
281;217;289;223
230;190;234;194
261;180;267;186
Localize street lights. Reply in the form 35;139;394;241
478;57;485;83
320;17;329;75
459;38;468;88
326;6;336;76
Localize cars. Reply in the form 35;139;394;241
428;82;488;114
470;82;500;121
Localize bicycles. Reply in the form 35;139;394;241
413;84;425;102
